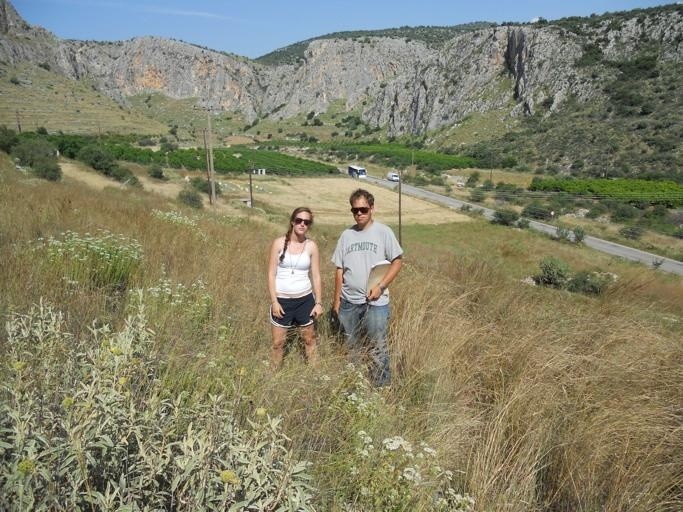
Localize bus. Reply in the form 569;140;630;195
347;165;367;181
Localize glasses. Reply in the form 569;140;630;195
293;216;312;226
351;205;371;214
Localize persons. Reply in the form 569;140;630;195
328;187;405;387
268;206;324;370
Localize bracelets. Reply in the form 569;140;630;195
314;300;323;306
376;281;387;292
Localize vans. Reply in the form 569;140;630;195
387;172;400;182
